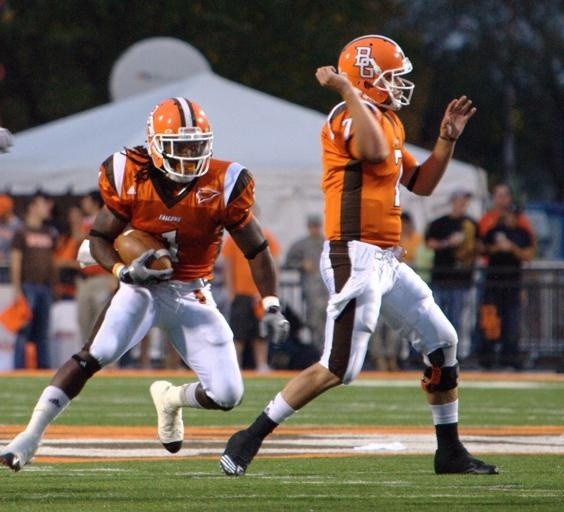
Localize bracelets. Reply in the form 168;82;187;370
438;135;458;143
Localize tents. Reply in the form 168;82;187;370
2;76;486;367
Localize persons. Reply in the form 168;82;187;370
0;127;16;154
221;203;330;375
219;34;498;476
0;96;290;474
0;184;165;373
370;183;537;373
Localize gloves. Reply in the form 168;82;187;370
119;248;174;288
258;305;291;346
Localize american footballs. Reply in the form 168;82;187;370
113;230;171;273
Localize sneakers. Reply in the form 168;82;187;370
148;379;185;455
433;448;500;476
1;433;40;474
219;429;262;479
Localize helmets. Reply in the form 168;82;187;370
337;34;416;112
145;96;214;184
